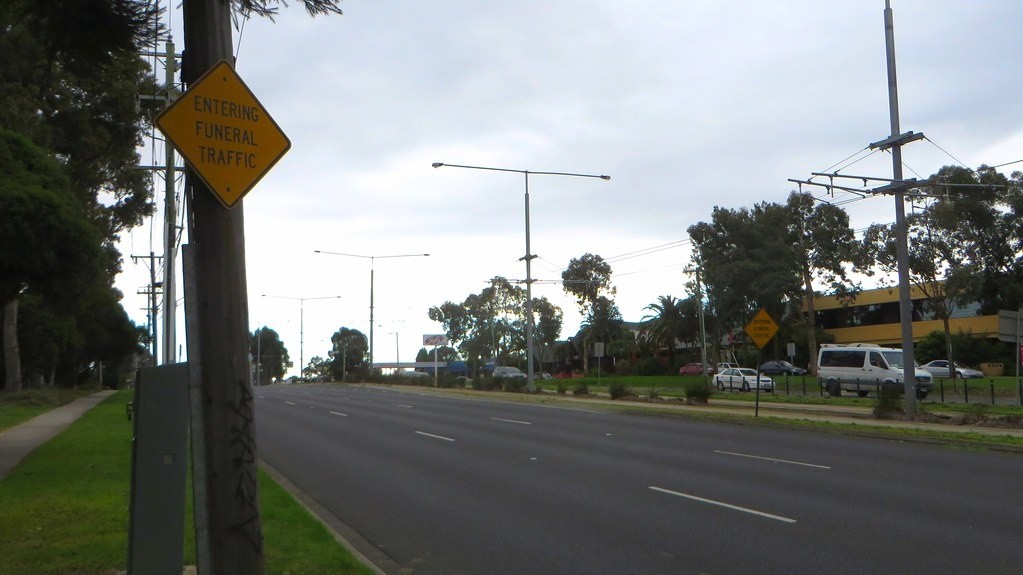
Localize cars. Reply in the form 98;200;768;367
759;361;807;375
715;362;738;373
922;360;984;379
712;368;775;392
679;363;714;376
492;366;527;379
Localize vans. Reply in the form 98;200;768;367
817;343;935;399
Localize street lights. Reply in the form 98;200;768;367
433;162;611;394
315;250;430;378
262;294;341;383
